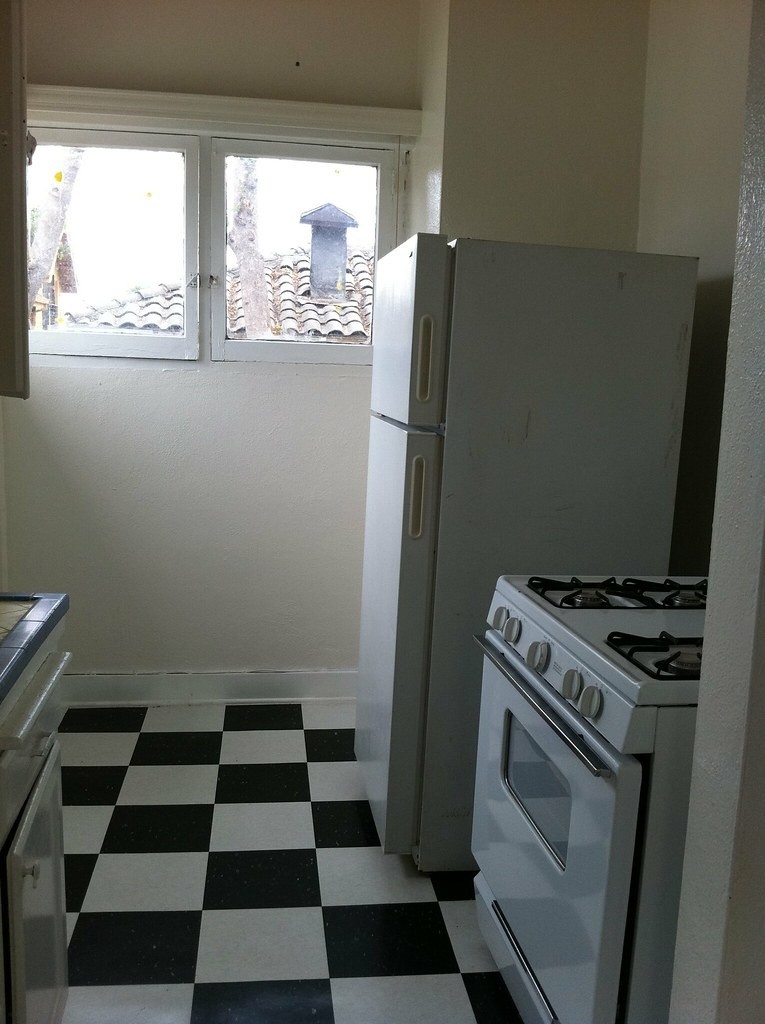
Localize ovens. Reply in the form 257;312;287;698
466;628;698;1023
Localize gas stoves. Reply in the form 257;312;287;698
485;574;715;705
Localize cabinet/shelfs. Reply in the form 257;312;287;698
1;590;75;1022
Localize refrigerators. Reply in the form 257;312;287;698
356;230;699;876
